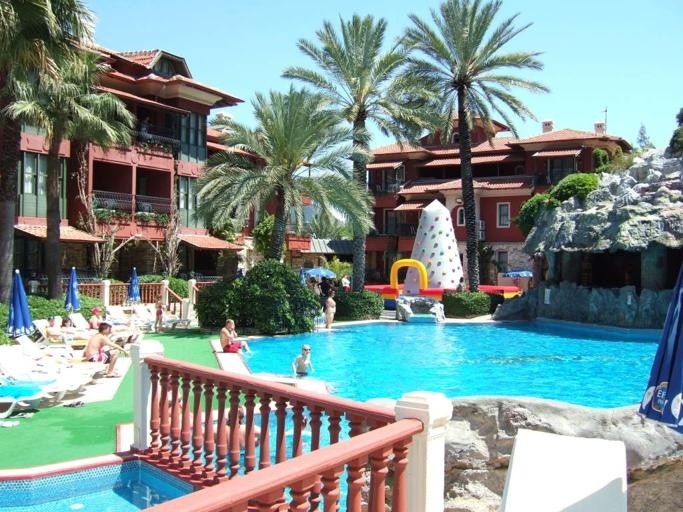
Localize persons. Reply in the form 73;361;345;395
58;319;93;337
89;307;117;330
46;316;88;342
341;273;351;292
323;289;336;331
153;300;164;333
304;274;335;301
456;277;466;293
96;325;139;350
290;344;313;380
220;319;249;356
82;323;129;378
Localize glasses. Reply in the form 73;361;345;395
303;349;310;351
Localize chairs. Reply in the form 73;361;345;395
0;302;191;418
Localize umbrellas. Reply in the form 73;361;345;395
5;269;35;339
636;264;683;435
127;266;141;303
62;266;81;311
301;267;336;280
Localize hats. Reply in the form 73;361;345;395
48;317;55;320
